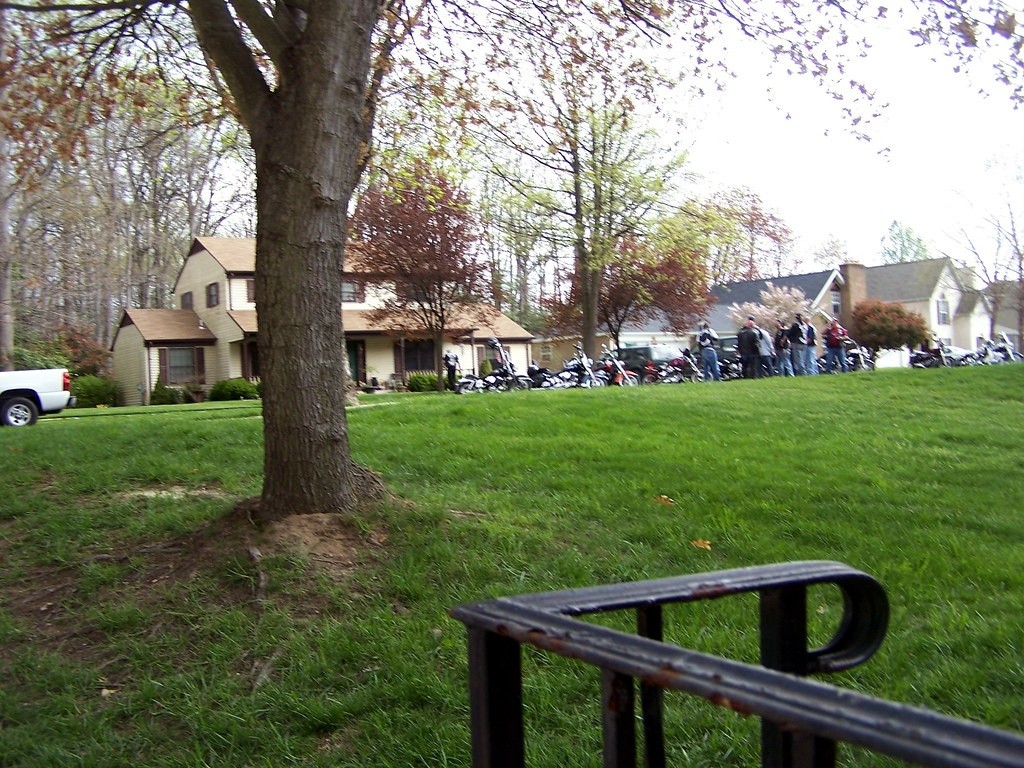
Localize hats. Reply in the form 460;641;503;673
746;320;754;329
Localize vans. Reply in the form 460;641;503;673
600;345;675;369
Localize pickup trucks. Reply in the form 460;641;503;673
0;369;77;429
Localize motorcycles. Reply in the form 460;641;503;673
816;336;874;373
453;337;742;396
909;332;1024;369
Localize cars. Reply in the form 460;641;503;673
691;336;743;362
941;346;969;359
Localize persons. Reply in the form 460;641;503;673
696;324;722;382
737;312;946;379
445;350;460;392
